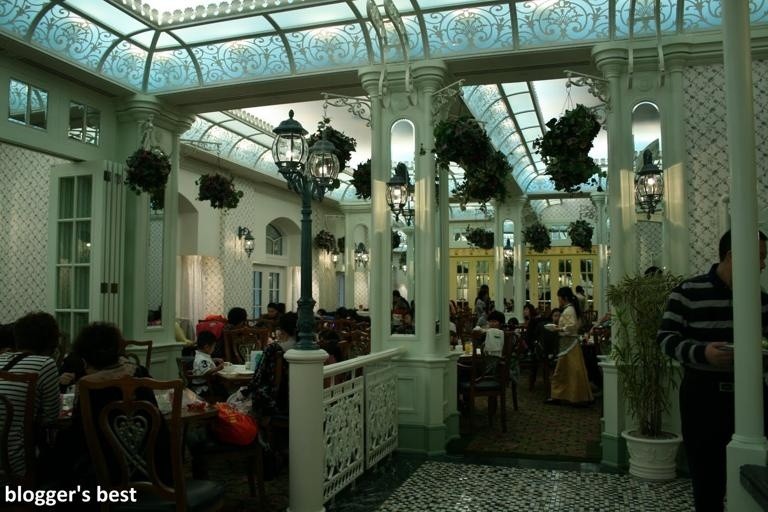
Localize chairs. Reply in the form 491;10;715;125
448;299;611;429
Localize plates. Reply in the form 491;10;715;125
238;371;255;375
724;343;768;353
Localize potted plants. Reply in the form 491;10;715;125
607;269;691;483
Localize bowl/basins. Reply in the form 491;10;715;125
544;325;557;330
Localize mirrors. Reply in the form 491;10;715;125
630;101;663;282
389;117;417;335
146;190;166;331
503;219;513;312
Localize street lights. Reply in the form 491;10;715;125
265;108;339;512
386;160;440;228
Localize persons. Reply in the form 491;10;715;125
656;228;768;511
66;319;170;504
58;348;87;394
0;310;64;501
221;284;611;407
190;328;229;405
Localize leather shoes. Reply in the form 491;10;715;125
544;398;559;404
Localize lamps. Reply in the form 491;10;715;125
502;240;512;262
385;160;408;222
400;175;415;226
331;250;339;268
634;177;661;221
239;226;255;259
